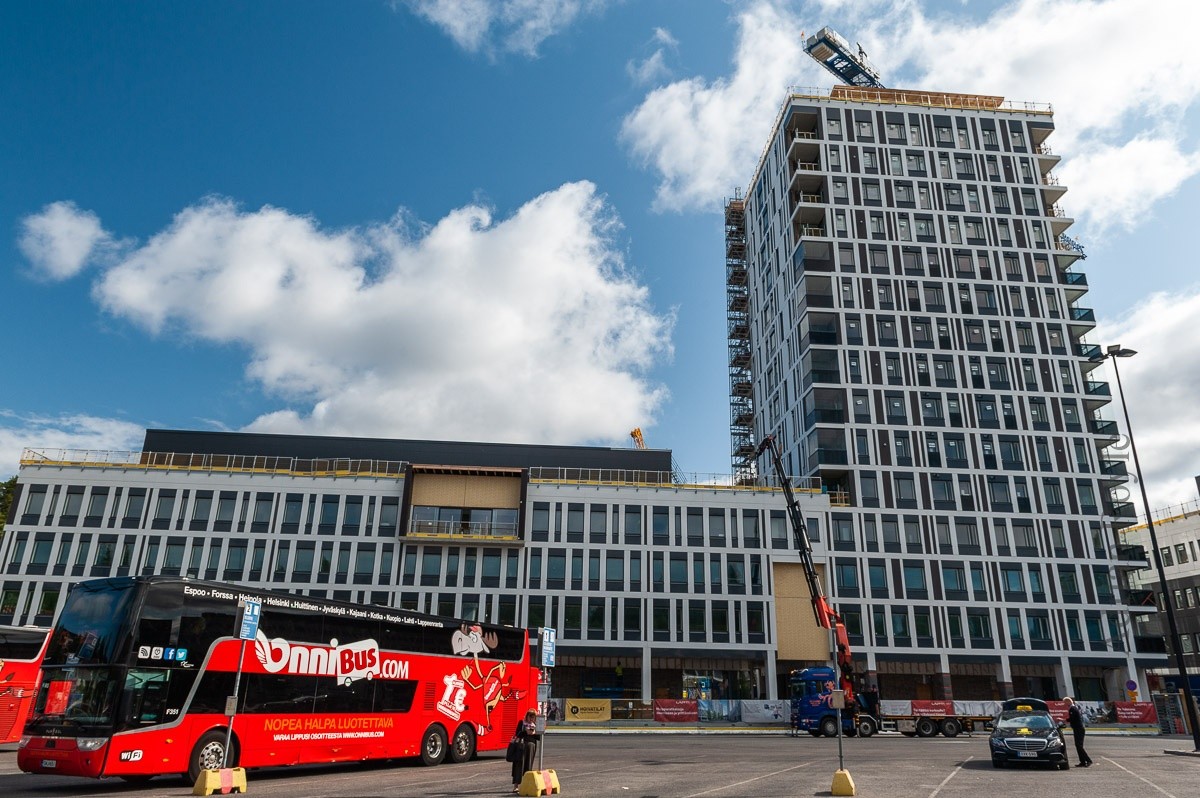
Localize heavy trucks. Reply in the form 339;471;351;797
788;667;996;737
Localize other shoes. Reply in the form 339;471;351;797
1085;760;1093;768
1075;763;1086;767
513;789;519;793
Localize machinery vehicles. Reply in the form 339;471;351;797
750;436;859;703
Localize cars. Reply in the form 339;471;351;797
989;697;1069;770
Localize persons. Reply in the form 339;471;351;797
512;709;538;793
1060;697;1093;768
546;702;562;722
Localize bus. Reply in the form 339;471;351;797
0;576;547;785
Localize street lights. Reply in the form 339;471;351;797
1088;343;1200;751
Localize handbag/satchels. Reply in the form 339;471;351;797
506;731;524;762
